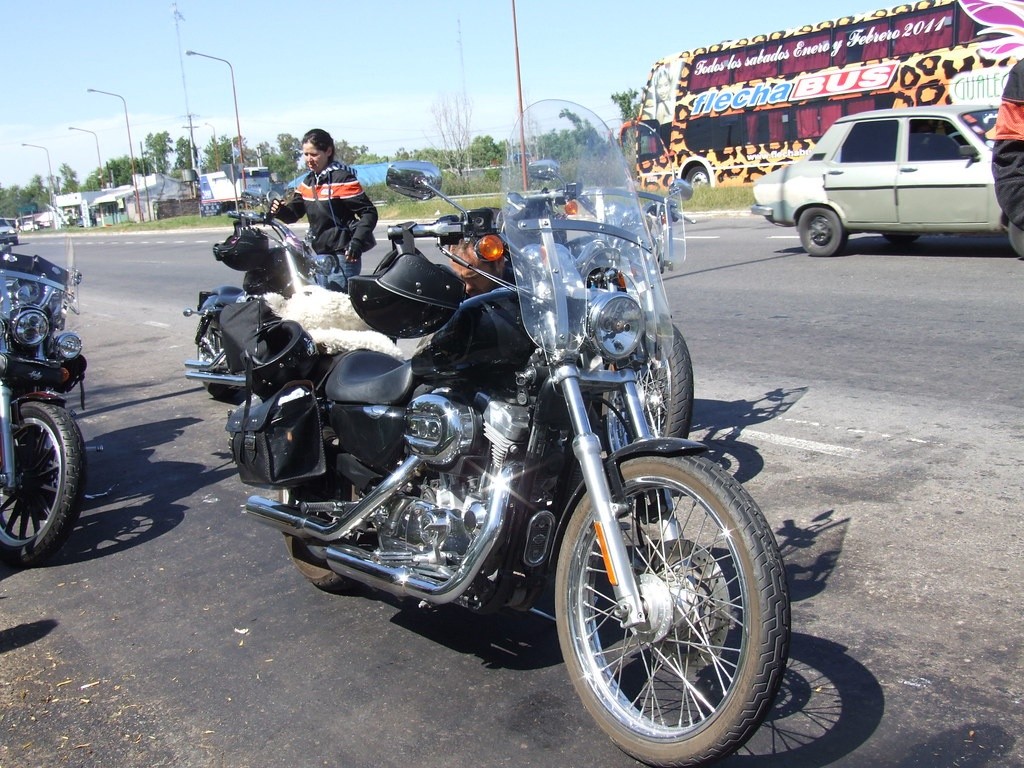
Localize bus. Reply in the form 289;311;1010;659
615;1;1024;193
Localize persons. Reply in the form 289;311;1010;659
654;69;674;126
265;129;378;293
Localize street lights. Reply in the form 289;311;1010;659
22;142;55;195
186;49;248;208
87;89;141;223
69;126;105;191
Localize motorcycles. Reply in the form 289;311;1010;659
1;193;88;568
183;212;355;404
227;100;789;768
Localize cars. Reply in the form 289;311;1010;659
750;101;1024;259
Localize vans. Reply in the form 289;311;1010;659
0;218;19;246
